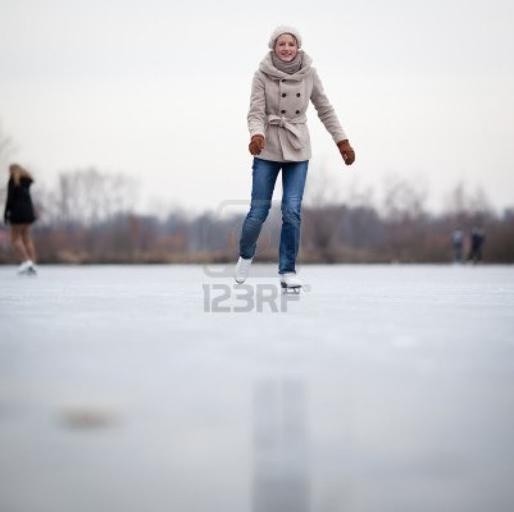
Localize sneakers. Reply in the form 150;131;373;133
279;272;302;288
235;254;254;283
17;259;36;274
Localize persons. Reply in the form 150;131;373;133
451;226;486;264
232;24;356;291
3;164;38;272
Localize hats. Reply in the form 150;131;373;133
268;24;301;50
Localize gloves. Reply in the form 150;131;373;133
249;134;266;154
337;139;355;165
